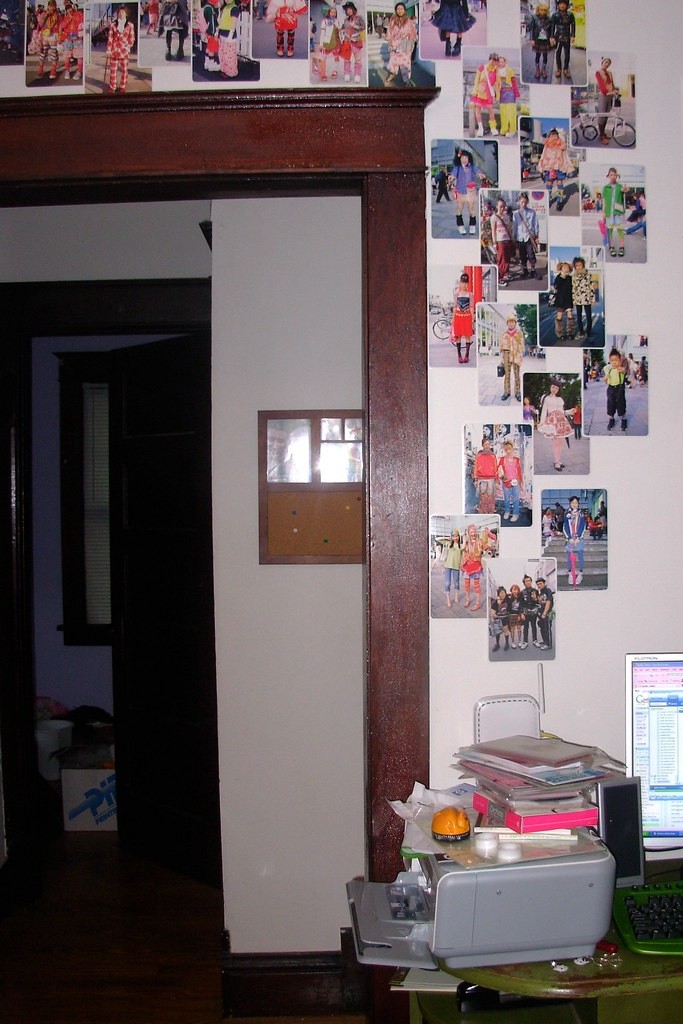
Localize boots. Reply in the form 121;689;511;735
545;185;565;210
598;121;612;144
445;36;462;56
277;31;295;57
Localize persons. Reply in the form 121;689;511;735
27;0;83;80
432;438;607;651
432;128;648;470
428;0;619;144
310;2;366;83
266;0;308;57
203;0;240;79
144;0;189;61
377;3;419;87
106;7;136;94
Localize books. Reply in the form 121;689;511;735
449;735;606;840
389;966;464;992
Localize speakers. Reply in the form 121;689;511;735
597;776;644;887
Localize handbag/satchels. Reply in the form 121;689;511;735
497;363;505;377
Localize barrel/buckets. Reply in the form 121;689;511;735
35;719;74;781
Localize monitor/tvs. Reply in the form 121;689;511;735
625;653;683;862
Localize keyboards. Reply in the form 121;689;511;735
613;880;683;956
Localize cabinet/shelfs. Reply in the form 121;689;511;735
411;857;683;1024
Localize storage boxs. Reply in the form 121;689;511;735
59;760;118;832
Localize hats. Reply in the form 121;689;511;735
450;529;459;535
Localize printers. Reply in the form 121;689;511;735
346;802;617;970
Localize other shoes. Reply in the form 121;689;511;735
107;85;126;93
559;331;596;341
502;393;522;403
456;214;477;235
164;48;185;60
385;73;414;88
493;640;552;652
320;71;362;83
36;64;82;82
477;121;516;138
503;514;522;523
447;600;482;610
457;353;469;363
497;268;542;287
203;56;241;80
567;571;583;585
553;461;565;471
535;63;571;80
609;246;626;257
607;418;629;430
